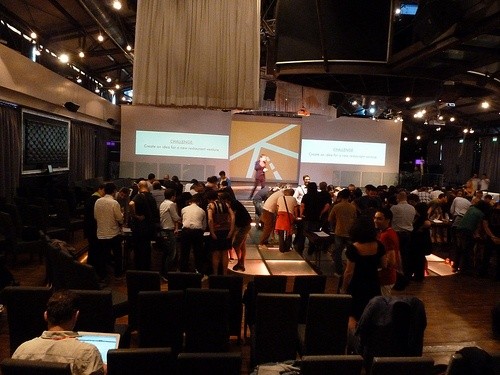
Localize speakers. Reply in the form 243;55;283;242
107;118;117;126
65;102;80;112
328;92;342;106
264;82;277;101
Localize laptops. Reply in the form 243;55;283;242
77;331;121;365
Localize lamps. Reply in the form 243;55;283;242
107;117;118;125
264;82;277;101
65;102;80;112
362;95;369;109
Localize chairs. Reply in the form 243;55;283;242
0;224;435;375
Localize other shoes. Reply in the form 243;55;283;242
115;275;126;280
233;260;240;271
160;270;169;282
261;242;273;247
240;259;245;272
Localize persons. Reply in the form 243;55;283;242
223;188;252;272
207;191;231;275
159;188;182;282
129;180;158;270
83;184;105;265
181;195;206;271
129;171;236;208
94;183;124;246
252;172;500;355
115;187;130;208
11;288;108;375
248;154;266;201
